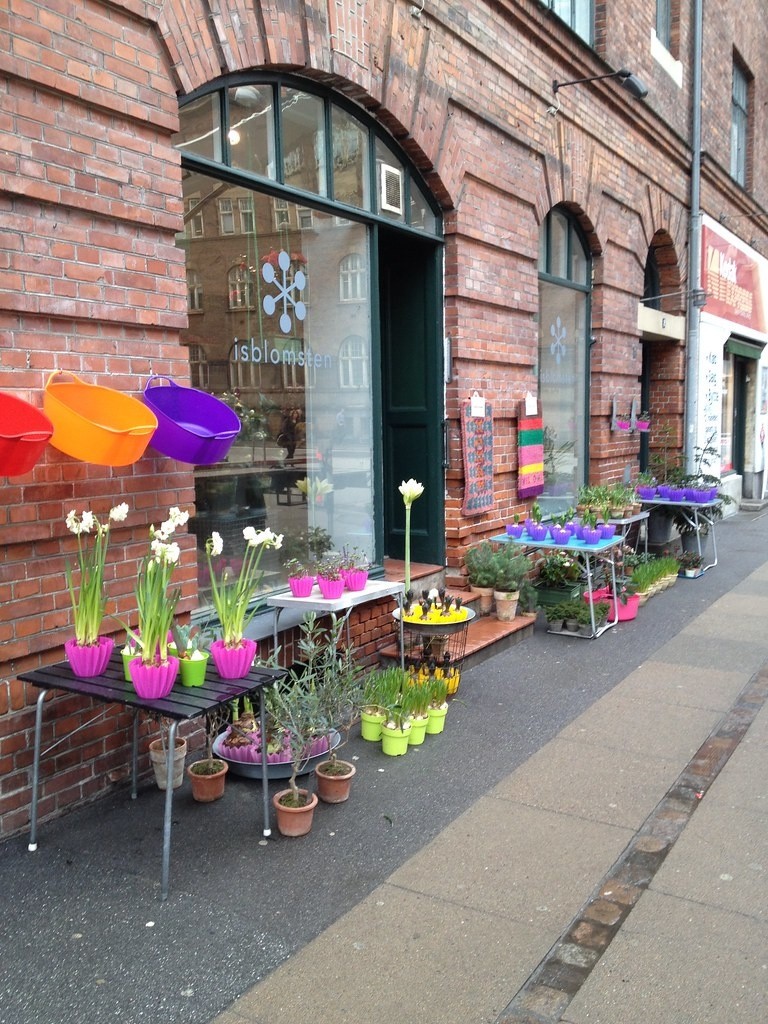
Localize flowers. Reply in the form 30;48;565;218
58;471;281;664
285;542;372;581
666;546;704;570
399;478;426;606
266;607;367;801
599;544;633;567
294;476;334;506
537;549;576;586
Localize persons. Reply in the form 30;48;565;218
336;408;348;444
282;410;299;467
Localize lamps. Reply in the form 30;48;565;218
553;68;649;98
640;286;714;309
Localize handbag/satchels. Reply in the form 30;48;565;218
276;433;288;448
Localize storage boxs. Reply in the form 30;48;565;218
535;582;580;608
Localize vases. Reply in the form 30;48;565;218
2;372;240;465
315;763;357;803
692;568;701;576
273;787;318;837
685;568;697;578
211;639;256;679
126;656;179;700
289;577;315;598
64;637;113;677
220;724;330;764
624;566;635;575
130;629;173;654
345;570;369;591
179;652;208;687
584;588;640;621
120;648;142;681
321;579;344;599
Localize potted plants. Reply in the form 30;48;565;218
658;419;723;501
185;704;231;803
494;468;656;544
464;541;526;621
149;712;187;790
616;412;629;429
636;555;681;606
663;430;734;560
636;411;651;429
543;600;609;637
360;589;467;757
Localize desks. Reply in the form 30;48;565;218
633;497;724;572
572;510;649;584
489;527;625;639
16;646;288;900
